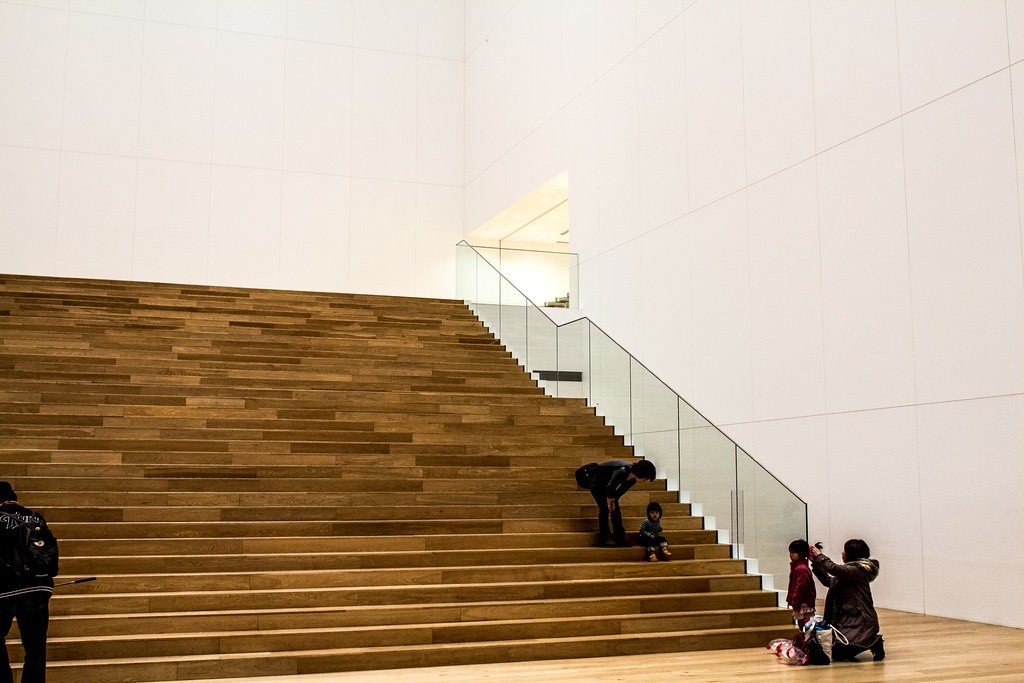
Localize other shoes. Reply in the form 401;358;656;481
663;550;672;560
648;554;658;562
870;637;885;661
615;541;629;547
599;539;608;548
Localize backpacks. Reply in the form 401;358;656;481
0;510;59;579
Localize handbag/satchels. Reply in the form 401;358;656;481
795;631;830;665
575;463;598;489
803;619;849;662
766;632;809;665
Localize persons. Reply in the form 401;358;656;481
810;539;894;661
785;540;823;643
588;457;658;547
0;480;60;683
639;501;672;563
